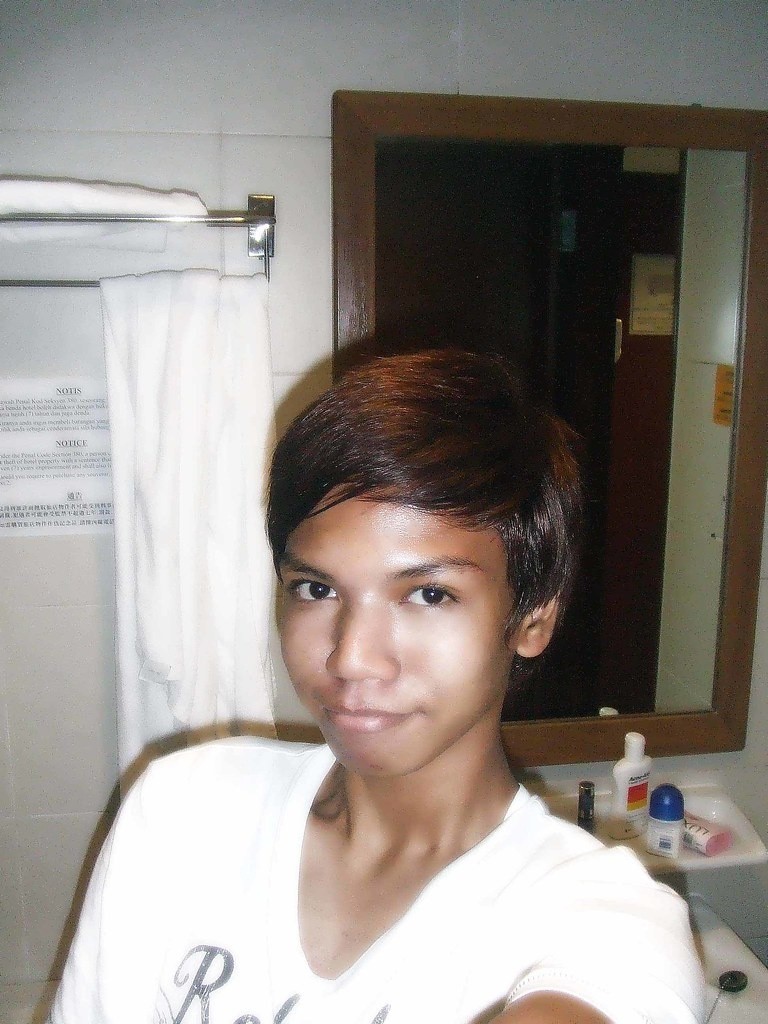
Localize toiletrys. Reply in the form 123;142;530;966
609;729;730;860
595;705;616;715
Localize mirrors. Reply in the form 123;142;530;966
330;91;768;768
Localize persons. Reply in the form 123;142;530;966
51;350;705;1024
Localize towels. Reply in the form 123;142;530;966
0;175;209;254
98;269;281;807
